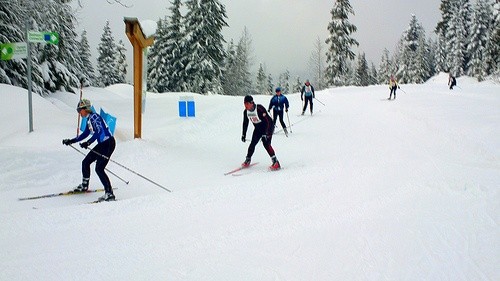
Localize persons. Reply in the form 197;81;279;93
388;75;400;100
62;99;116;203
268;87;289;135
241;95;281;171
447;73;456;89
301;81;315;116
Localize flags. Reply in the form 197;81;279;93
78;105;117;135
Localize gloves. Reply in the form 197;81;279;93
81;141;88;149
268;110;270;112
285;108;288;112
63;139;73;146
301;97;303;101
313;94;315;98
242;135;246;142
265;132;270;141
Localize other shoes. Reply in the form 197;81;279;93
388;98;391;100
74;183;88;192
311;112;312;115
98;193;115;201
242;160;250;167
269;160;280;171
302;112;304;115
394;95;396;99
283;128;288;134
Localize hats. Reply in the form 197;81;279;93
306;82;309;85
276;88;281;91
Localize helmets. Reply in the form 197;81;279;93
77;99;91;108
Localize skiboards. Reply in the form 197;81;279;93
18;187;120;210
223;162;284;176
